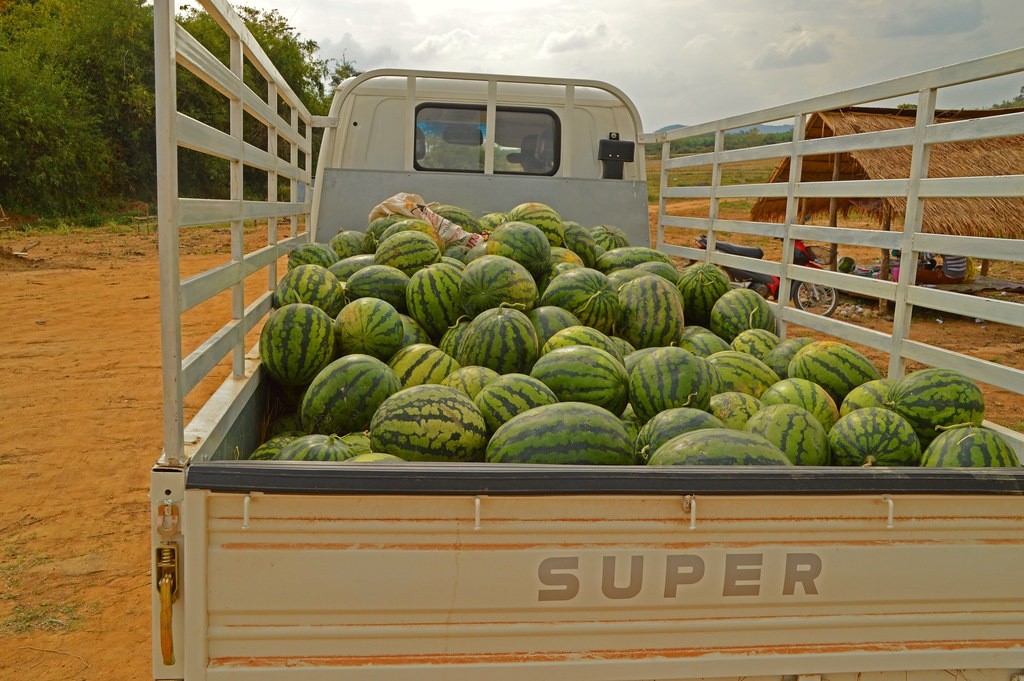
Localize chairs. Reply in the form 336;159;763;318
534;127;554;173
416;126;426;159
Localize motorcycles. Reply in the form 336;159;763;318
682;234;839;317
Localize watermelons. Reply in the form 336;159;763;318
247;201;1018;467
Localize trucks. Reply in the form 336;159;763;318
151;1;1024;681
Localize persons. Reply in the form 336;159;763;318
516;135;553;175
916;252;967;286
